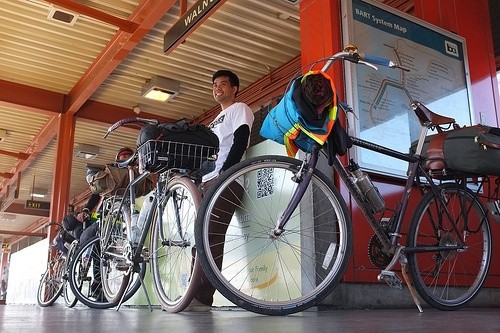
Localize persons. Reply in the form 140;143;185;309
162;69;255;313
76;147;138;303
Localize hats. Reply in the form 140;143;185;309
118;150;132;156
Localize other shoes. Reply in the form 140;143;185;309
161;297;212;312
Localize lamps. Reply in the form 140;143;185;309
140;75;180;103
30;188;48;199
75;144;99;160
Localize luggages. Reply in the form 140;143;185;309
411;124;500;178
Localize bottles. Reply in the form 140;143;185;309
347;159;386;213
131;210;141;246
137;196;154;230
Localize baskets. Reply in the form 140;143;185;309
136;140;220;177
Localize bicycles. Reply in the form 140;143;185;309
193;44;493;315
36;117;222;314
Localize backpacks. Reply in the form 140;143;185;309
135;118;220;170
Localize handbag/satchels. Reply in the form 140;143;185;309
88;163;157;198
